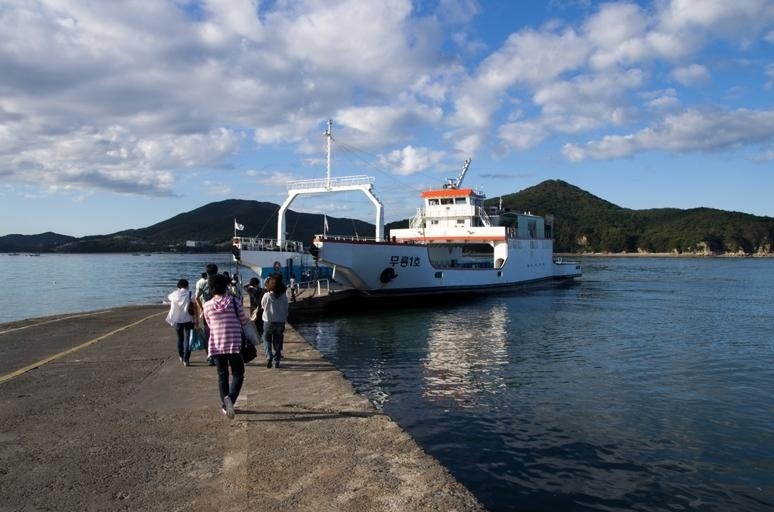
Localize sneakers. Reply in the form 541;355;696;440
207;356;217;365
178;356;189;366
275;361;279;368
222;396;235;419
266;355;273;368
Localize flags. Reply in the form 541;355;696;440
325;217;328;231
234;221;244;231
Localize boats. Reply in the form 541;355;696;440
231;116;584;319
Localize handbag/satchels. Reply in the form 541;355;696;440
189;328;205;350
188;302;195;315
241;339;257;362
251;304;263;335
242;321;262;345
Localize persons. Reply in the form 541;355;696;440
288;274;298;304
200;274;244;419
257;276;288;368
183;263;272;366
299;270;311;282
165;279;196;367
